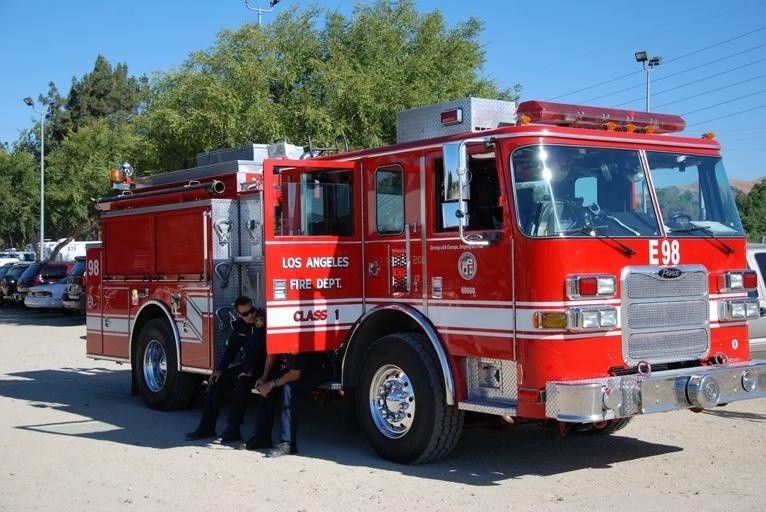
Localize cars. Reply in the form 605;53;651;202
0;250;85;310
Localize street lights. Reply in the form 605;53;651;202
23;96;54;262
634;50;663;112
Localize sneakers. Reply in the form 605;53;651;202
265;440;292;459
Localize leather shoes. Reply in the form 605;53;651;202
185;428;216;441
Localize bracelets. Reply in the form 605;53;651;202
271;378;278;386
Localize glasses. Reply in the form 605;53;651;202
237;306;255;317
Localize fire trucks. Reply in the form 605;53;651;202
84;96;762;463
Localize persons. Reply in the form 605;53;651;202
183;293;258;444
239;311;265;376
246;349;325;459
